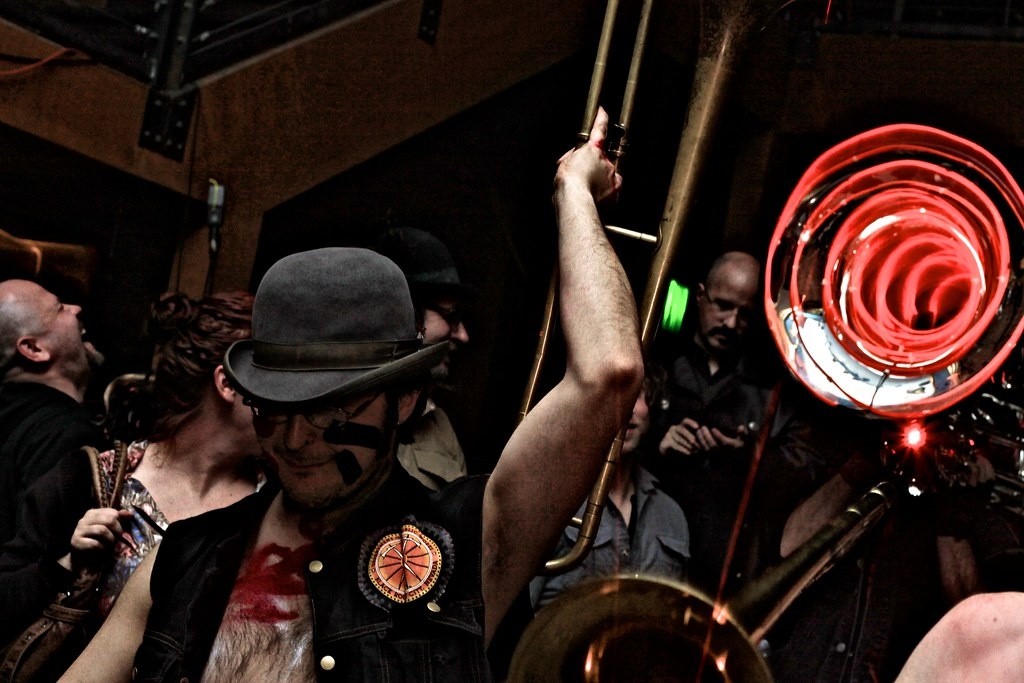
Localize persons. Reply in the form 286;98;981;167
0;243;1024;683
57;104;647;683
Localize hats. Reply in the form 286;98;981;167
223;248;452;405
359;228;481;313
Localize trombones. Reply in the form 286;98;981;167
511;0;795;580
505;468;913;683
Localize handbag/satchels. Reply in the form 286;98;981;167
0;440;128;683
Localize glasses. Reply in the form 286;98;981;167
421;303;464;330
242;383;392;432
